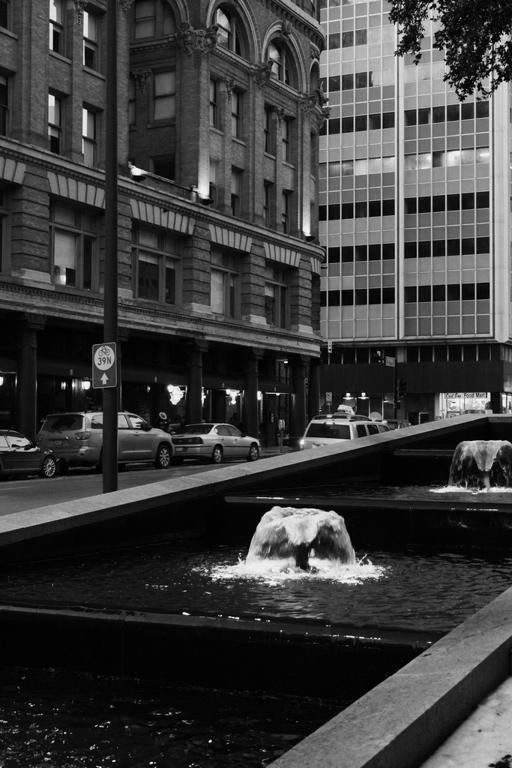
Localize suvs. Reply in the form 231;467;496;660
299;411;392;453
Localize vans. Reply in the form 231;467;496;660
34;408;179;471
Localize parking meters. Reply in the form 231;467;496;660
278;418;286;455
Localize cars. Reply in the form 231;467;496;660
0;425;65;479
165;421;263;465
383;418;412;430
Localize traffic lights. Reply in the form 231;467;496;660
399;377;409;399
